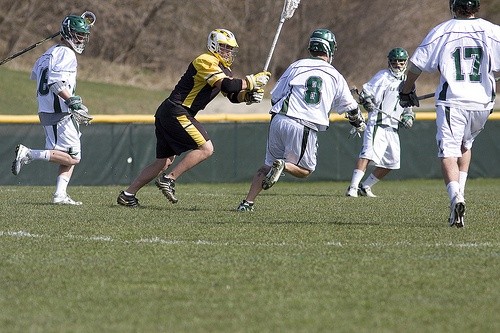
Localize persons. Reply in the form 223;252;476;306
236;28;368;213
11;15;93;205
346;47;415;198
401;0;500;228
116;28;272;208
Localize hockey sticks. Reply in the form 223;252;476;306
73;109;94;125
349;88;403;123
263;0;301;73
399;76;500;107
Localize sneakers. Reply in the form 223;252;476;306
358;183;377;197
116;190;140;207
346;185;358;197
52;193;82;205
237;200;254;212
262;159;285;190
154;173;178;204
12;144;31;175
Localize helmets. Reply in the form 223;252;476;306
387;47;408;77
449;0;480;18
308;29;337;65
207;29;239;68
59;16;90;54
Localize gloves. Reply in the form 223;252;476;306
399;83;419;107
64;96;88;114
245;72;271;91
349;114;367;134
400;112;416;129
363;96;375;112
239;89;264;105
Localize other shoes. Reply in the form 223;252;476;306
450;194;466;228
